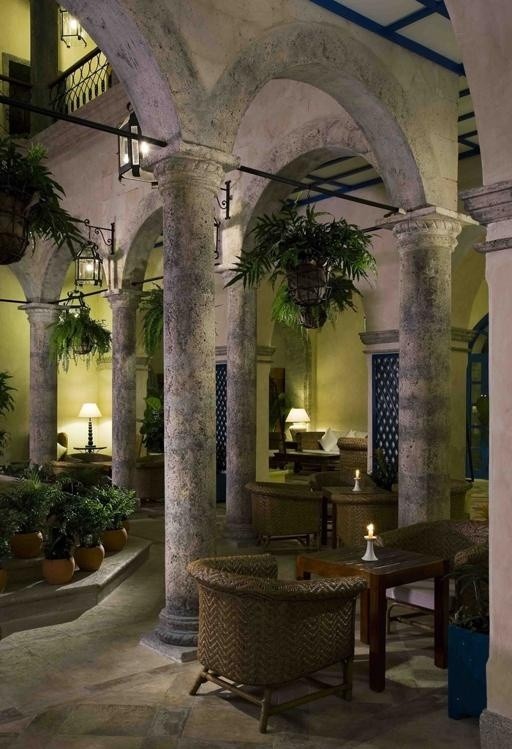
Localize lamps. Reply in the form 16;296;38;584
285;407;311;438
118;100;233;220
59;7;87;48
74;403;107;456
73;219;115;286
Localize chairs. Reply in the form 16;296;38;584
311;468;379;490
271;429;371;482
321;492;397;551
363;514;488;637
186;551;365;737
244;479;325;552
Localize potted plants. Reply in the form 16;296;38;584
225;194;386;330
0;469;139;588
0;131;84;266
47;304;111;367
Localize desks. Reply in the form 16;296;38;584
294;543;453;695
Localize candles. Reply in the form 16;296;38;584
363;520;378;537
352;468;365;481
